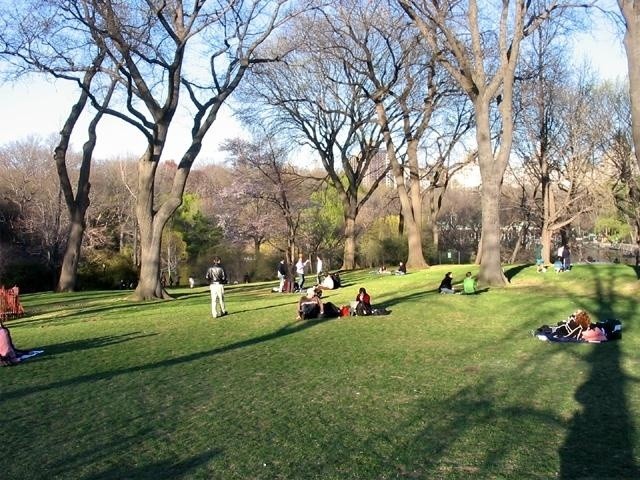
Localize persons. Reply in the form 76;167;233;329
560;312;592;341
393;260;406;276
272;251;373;319
0;320;17;366
379;265;388;274
438;272;463;295
205;255;228;319
462;271;479;295
160;268;194;289
535;239;572;274
